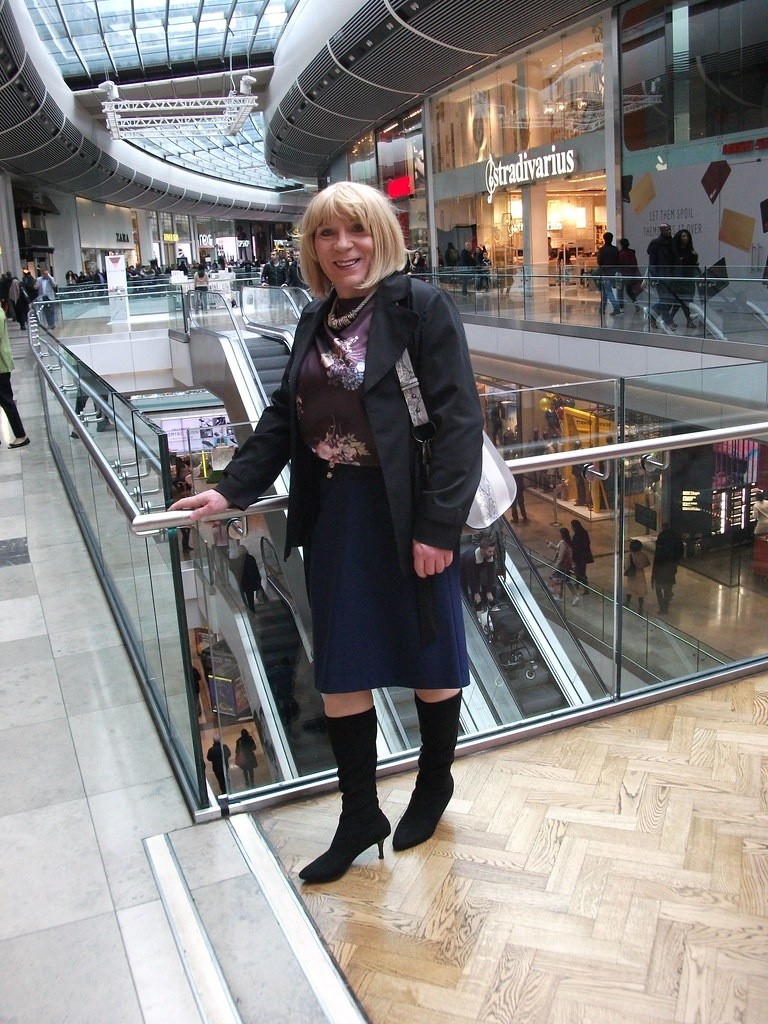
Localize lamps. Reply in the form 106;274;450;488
97;0;118;99
238;2;255;96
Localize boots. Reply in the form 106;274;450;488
392;690;463;851
298;706;393;882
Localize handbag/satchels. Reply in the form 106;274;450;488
422;426;518;534
554;554;572;571
256;586;267;602
615;271;623;289
624;563;636;577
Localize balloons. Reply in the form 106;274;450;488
539;392;575;429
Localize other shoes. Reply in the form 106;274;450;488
553;596;563;602
8;437;30;449
648;315;659;330
634;302;642;312
572;596;579;605
97;423;118;432
581;591;589;595
619;307;624;313
47;325;55;330
599;309;605;314
70;432;94;439
686;318;697;328
21;326;26;330
664;321;678;331
574;584;579;588
657;608;667;614
610;308;620;315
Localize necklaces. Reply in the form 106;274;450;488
328;287;378;330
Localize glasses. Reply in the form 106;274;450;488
270;253;276;256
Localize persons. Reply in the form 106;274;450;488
206;734;231;793
510;473;529;523
460;519;507;637
545;527;579;606
652;530;683;615
0;308;31;449
623;540;650;614
568;520;594;595
167;183;486;883
493;402;615;511
0;267;108;329
169;455;262;613
398;241;514;299
547;224;699;331
233;729;258;787
126;250;309;325
751;491;768;537
192;667;202;716
